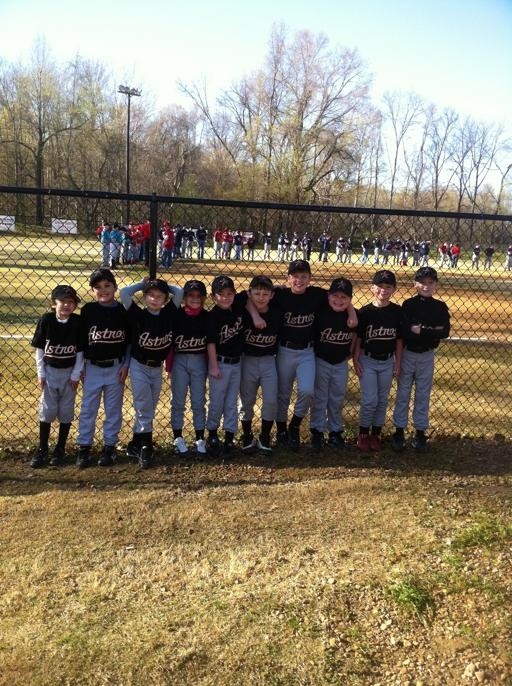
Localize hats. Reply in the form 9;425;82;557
371;269;396;287
414;266;440;283
327;276;353;298
249;275;274;289
182;279;206;296
210;275;237;293
89;269;114;285
51;284;83;304
142;279;170;295
288;259;312;273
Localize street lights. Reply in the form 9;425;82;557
117;82;142;224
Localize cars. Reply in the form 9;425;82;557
233;230;253;244
97;225;139;245
191;229;208;246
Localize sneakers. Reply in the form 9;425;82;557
49;445;68;466
29;445;49;467
172;435;189;455
73;444;94;468
138;446;155;469
97;444;122;468
191;438;209;460
125;440;141;458
391;431;409;451
207;426;384;457
412;435;430;453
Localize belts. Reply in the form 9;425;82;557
130;354;161;367
216;355;240;365
363;349;396;362
88;357;123;368
403;345;433;354
278;340;313;351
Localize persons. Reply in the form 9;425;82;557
239;273;284;451
119;276;184;469
391;267;450;453
204;275;249;458
164;280;209;455
352;269;404;453
243;260;359;453
313;276;359;449
75;267;131;467
31;284;86;467
